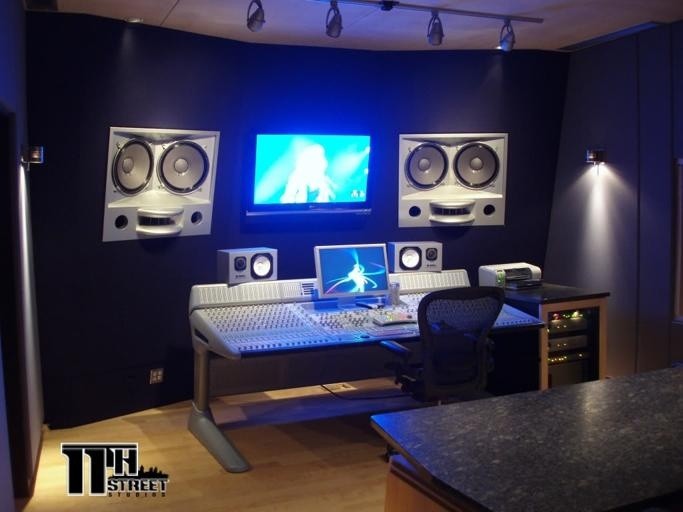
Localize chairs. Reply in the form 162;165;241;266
381;285;505;405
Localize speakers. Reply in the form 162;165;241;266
102;126;220;242
387;241;443;272
398;132;508;229
216;247;278;284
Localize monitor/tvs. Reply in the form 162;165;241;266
246;129;373;217
314;243;392;312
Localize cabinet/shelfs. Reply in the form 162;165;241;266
504;278;611;389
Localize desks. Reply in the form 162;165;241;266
368;364;683;511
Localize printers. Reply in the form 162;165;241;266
479;262;543;292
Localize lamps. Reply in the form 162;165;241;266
324;0;344;38
583;147;607;167
499;19;516;53
21;145;46;172
245;0;266;33
426;11;445;47
